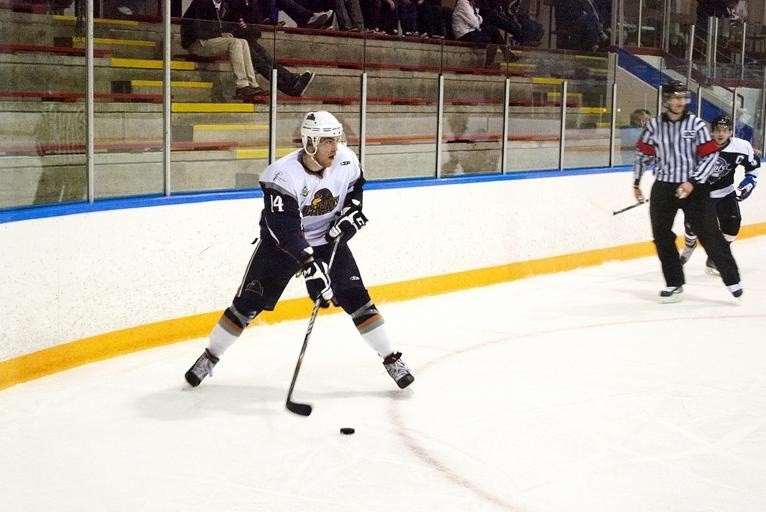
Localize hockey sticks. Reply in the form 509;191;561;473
288;240;342;416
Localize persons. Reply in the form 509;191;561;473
268;1;637;69
570;114;605;171
180;1;315;97
12;1;146;16
445;99;504;177
633;80;745;299
693;0;749;65
184;109;415;388
619;109;653;164
679;113;762;270
27;73;95;207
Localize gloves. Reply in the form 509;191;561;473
303;260;333;305
736;177;756;201
328;208;367;244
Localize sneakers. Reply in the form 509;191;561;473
679;242;697;266
728;284;743;298
659;284;684;297
185;347;220;386
382;352;414;389
704;257;721;273
307;9;446;40
237;86;270;97
293;71;315;97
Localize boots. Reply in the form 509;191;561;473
501;46;522;63
485;51;501;68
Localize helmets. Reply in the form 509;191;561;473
710;114;734;131
300;110;344;156
662;80;692;107
